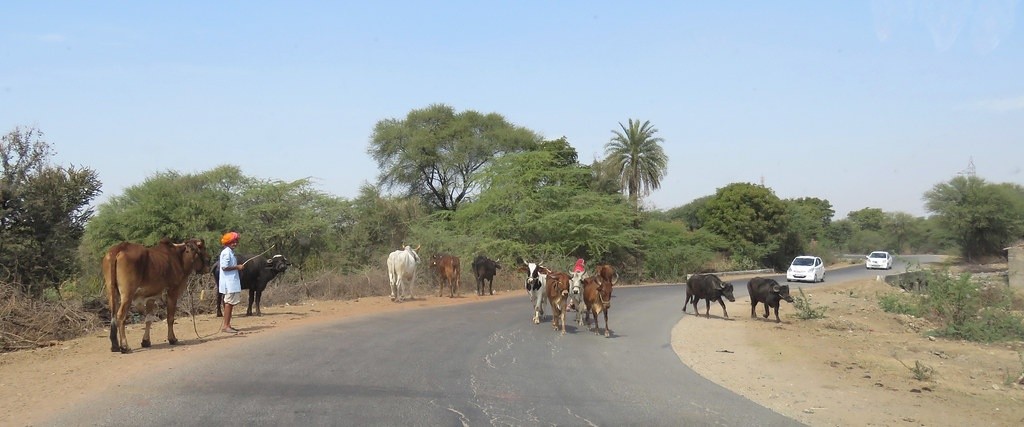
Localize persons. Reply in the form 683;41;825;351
218;232;244;332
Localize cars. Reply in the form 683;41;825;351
787;255;826;283
865;250;893;269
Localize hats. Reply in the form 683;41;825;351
222;232;240;246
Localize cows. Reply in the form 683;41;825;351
522;258;619;338
386;244;421;303
747;277;794;325
682;274;735;318
437;254;461;298
211;254;292;317
471;256;502;296
102;238;211;354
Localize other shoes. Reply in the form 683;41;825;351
222;325;240;333
